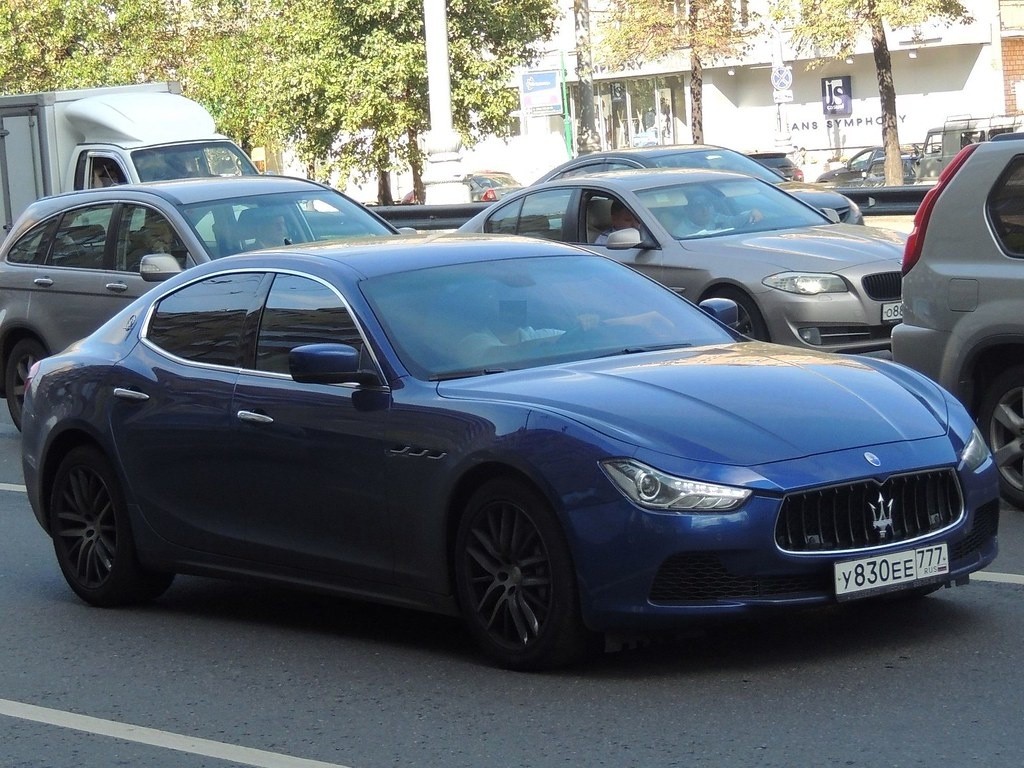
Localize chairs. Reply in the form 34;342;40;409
586;199;615;243
500;208;550;233
57;224;105;269
238;208;259;248
94;170;119;187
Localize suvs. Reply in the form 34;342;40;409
738;151;804;186
891;133;1024;513
0;175;419;432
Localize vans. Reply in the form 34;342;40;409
914;116;1024;183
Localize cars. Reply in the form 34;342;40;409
22;231;1001;671
438;166;918;359
402;171;522;207
528;145;865;233
813;144;932;188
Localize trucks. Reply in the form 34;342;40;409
0;80;275;250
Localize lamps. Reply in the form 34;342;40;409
846;55;855;64
728;66;735;76
909;49;918;58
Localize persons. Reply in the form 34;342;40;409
675;194;762;237
242;212;285;252
594;200;641;244
451;279;674;371
